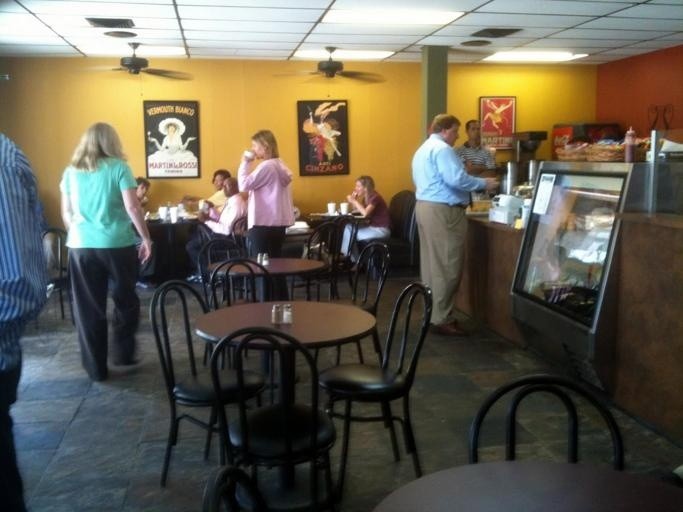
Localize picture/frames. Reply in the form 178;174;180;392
143;101;201;179
480;96;516;151
296;100;350;177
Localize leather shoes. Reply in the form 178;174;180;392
430;323;470;337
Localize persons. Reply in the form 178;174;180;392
451;119;496;178
237;130;293;301
59;123;151;383
131;176;159;291
148;118;197;175
411;115;500;337
188;170;232;272
334;175;390;272
185;178;246;284
0;133;47;512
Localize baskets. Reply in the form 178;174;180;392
554;147;584;160
584;144;643;161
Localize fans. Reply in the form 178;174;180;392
273;55;386;93
75;48;193;88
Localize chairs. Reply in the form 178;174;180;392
233;217;250;250
201;465;272;512
466;373;627;471
149;279;269;491
289;221;340;302
197;237;248;372
207;325;335;508
209;258;273;372
387;189;418;268
330;214;357;301
35;226;69;327
314;241;390;373
317;280;434;503
